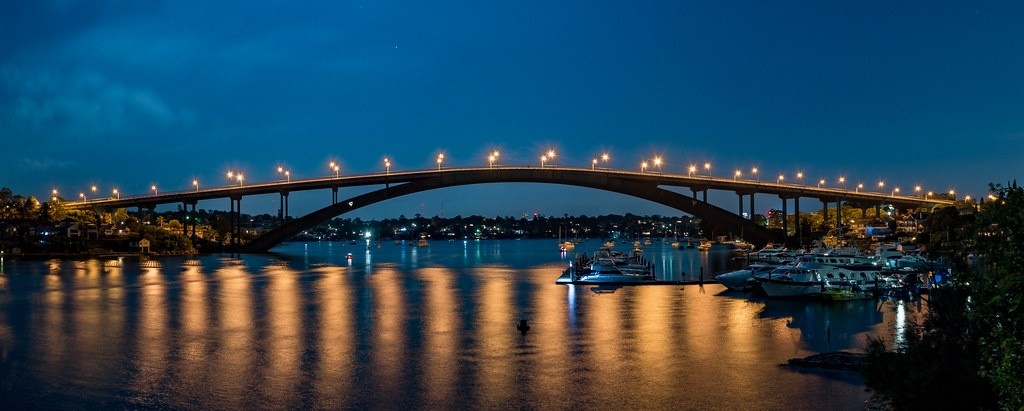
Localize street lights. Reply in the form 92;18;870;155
286;171;290;182
489;155;495;168
237;174;243;187
776;175;784;185
540;156;547;168
705;164;712;178
733;171;741;180
592;159;597;170
80;193;87;203
688;167;696;177
385;162;390;174
752;168;760;182
193;181;199;192
798;173;805;186
817;176;900;197
112;190;120;200
152;185;158;196
334;166;339;179
641;163;648;174
437;159;442;170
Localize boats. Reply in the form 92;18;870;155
419;239;428;246
715;247;969;295
